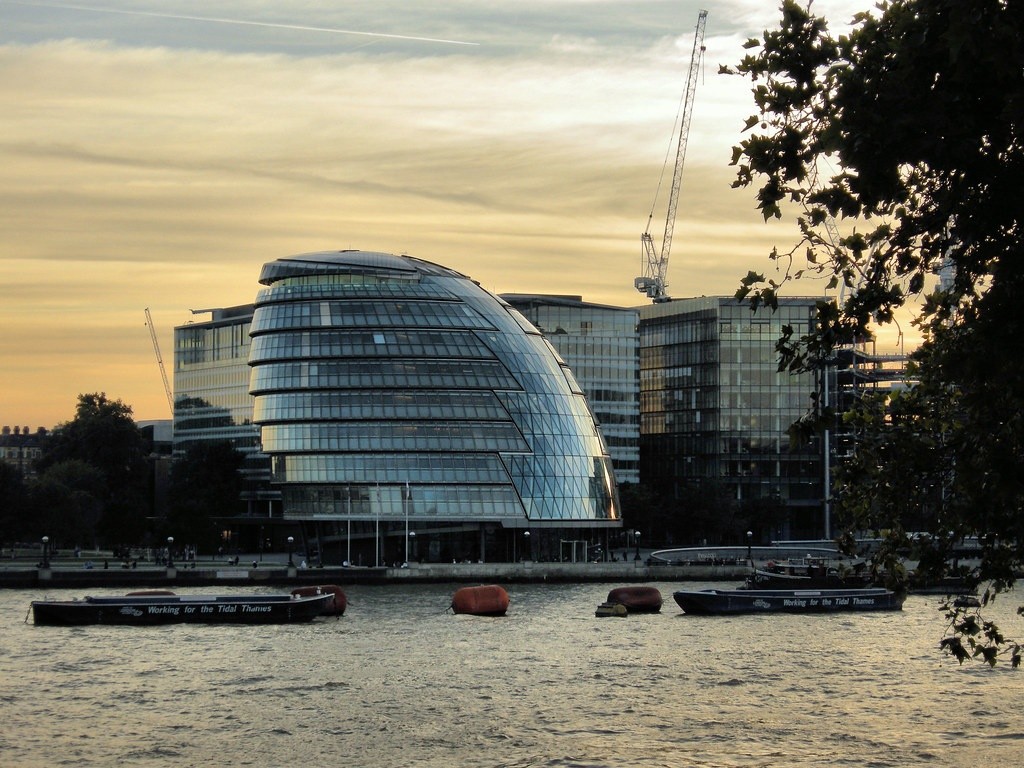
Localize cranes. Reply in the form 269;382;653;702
634;6;711;300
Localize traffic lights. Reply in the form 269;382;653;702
673;586;904;613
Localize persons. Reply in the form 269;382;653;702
10;545;409;571
552;547;781;569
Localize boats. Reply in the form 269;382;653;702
29;586;339;626
742;552;980;595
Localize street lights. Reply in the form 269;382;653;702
167;537;175;567
634;530;642;561
746;530;754;559
523;529;532;563
41;536;51;570
410;531;417;561
287;537;296;569
